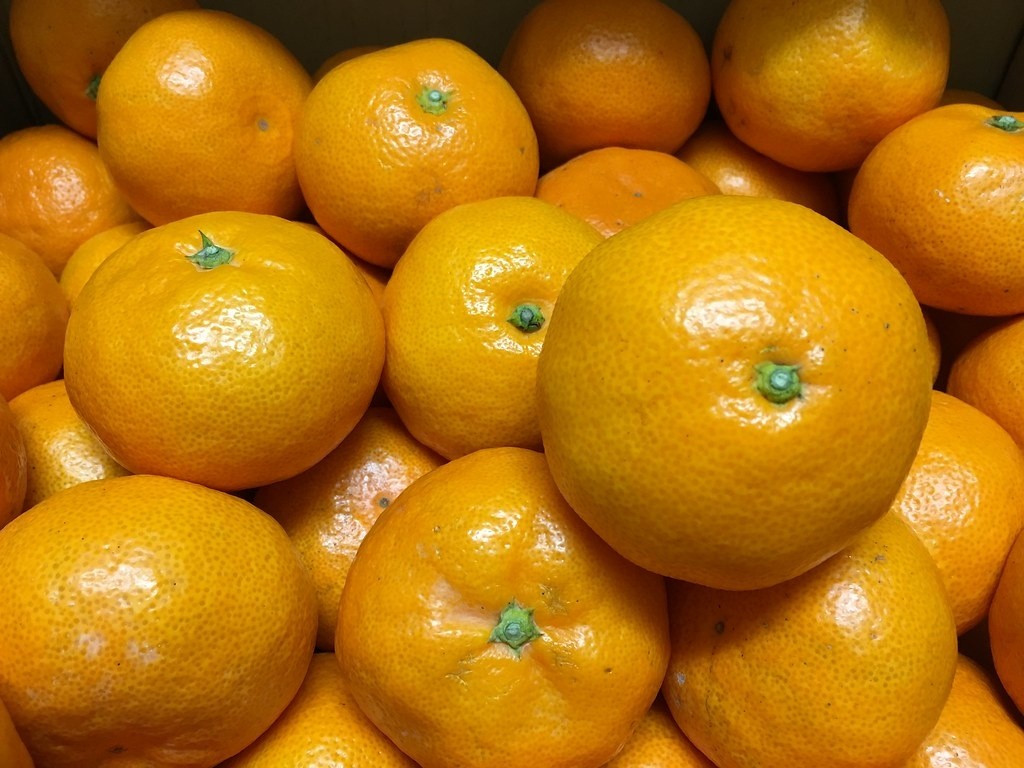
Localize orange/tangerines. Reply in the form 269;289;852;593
0;0;1024;768
